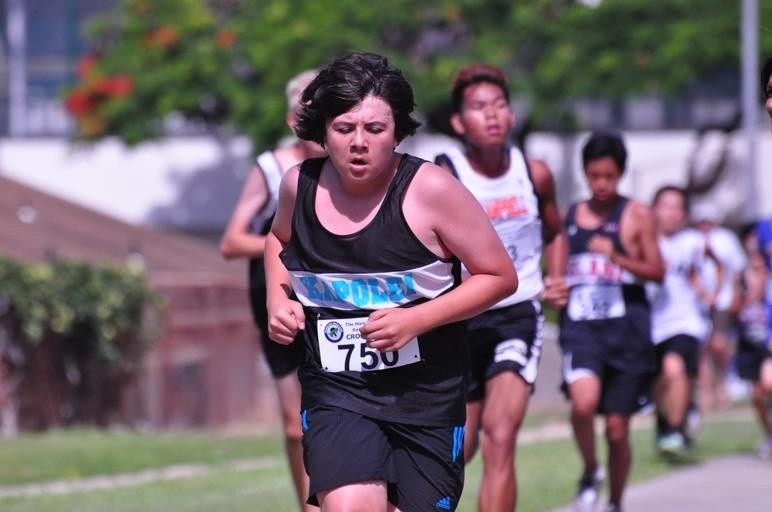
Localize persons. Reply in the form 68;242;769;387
262;51;518;512
553;128;666;512
220;68;328;511
431;67;569;512
643;185;772;460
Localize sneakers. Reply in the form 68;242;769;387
575;466;620;511
654;404;701;458
